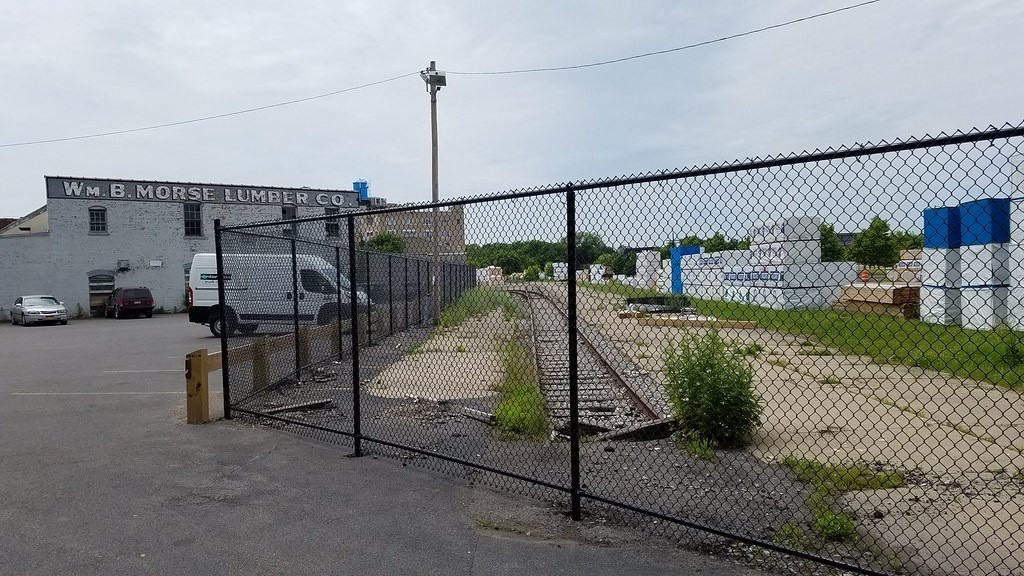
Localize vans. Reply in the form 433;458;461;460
188;253;377;339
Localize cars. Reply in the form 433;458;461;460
10;295;69;327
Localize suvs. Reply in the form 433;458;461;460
104;285;155;319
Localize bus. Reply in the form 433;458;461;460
898;259;924;270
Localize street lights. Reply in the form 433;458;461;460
422;61;447;327
